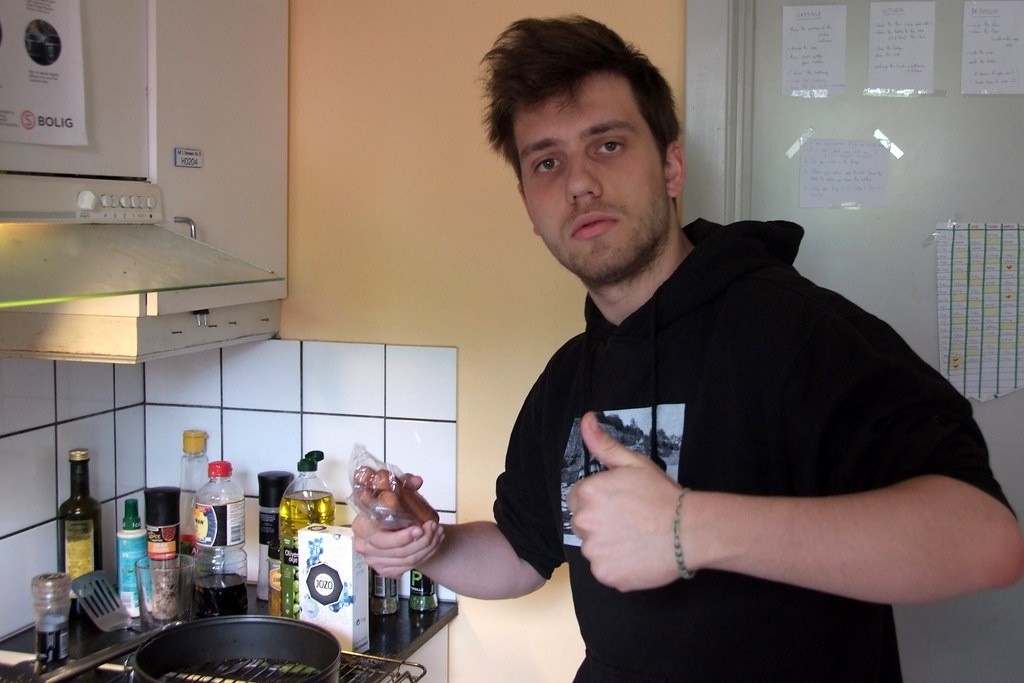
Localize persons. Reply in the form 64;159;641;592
351;14;1023;683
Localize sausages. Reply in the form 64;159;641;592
354;466;439;532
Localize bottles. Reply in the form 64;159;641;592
193;460;249;618
115;498;149;618
56;448;103;619
177;430;215;578
408;568;439;614
370;567;400;616
143;486;181;562
30;572;72;678
256;470;294;600
266;544;282;616
279;450;336;622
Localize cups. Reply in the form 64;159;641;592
136;553;196;634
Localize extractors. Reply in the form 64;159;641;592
0;169;285;308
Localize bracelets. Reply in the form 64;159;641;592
674;488;695;580
